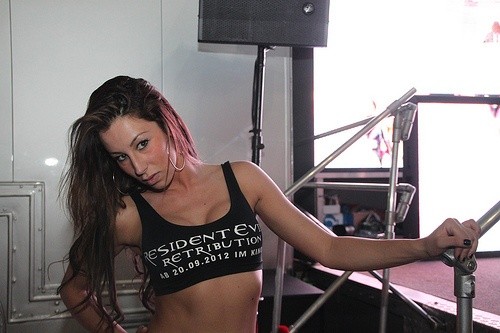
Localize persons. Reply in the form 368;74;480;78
58;75;481;333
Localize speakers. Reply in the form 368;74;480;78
199;0;329;47
